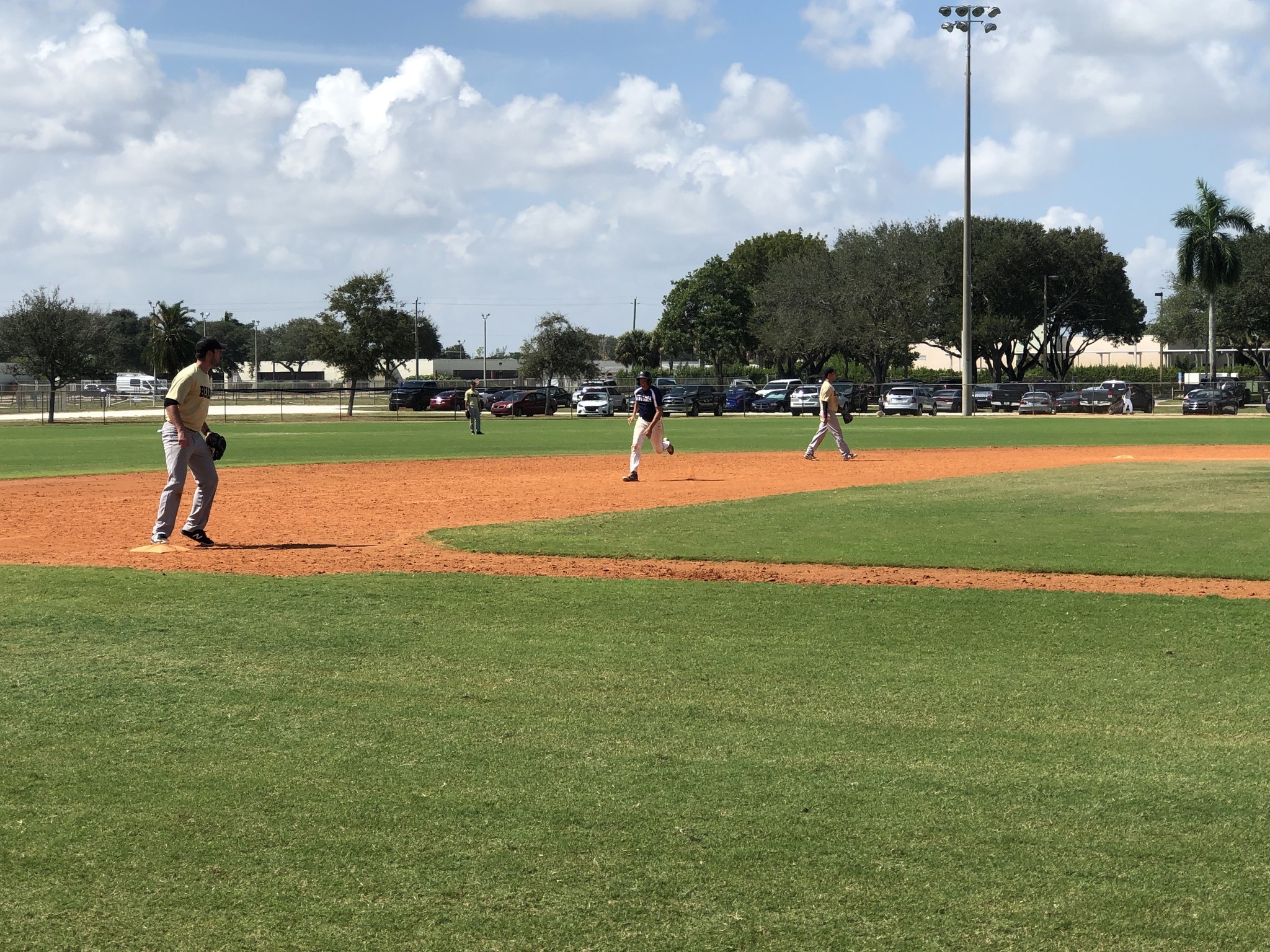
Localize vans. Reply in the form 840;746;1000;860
114;373;169;400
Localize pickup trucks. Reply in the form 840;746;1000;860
990;381;1034;413
389;381;455;412
1079;380;1155;414
1034;380;1078;401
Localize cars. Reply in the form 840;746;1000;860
627;378;978;417
82;387;109;397
430;391;484;411
1182;389;1239;415
1018;391;1056;415
84;384;111;395
971;385;1000;409
1265;393;1270;414
1204;377;1251;409
475;379;627;417
1054;392;1083;413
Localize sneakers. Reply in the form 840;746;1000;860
622;472;639;482
150;533;170;545
663;438;674;455
804;454;819;461
842;453;858;460
180;529;215;546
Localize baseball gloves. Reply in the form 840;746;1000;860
465;411;471;419
842;410;853;424
206;433;226;461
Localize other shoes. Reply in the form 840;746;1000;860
476;431;485;434
471;432;475;435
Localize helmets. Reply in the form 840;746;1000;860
636;371;653;386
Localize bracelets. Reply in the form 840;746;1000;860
203;428;209;435
465;408;468;410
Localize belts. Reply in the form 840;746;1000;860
166;420;200;433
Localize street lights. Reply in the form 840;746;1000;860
939;4;1001;416
1154;292;1163;395
1043;275;1060;381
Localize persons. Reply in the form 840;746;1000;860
464;381;485;435
1221;381;1229;390
803;366;858;462
149;336;226;548
1121;384;1134;415
621;370;674;483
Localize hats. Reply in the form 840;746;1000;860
196;336;227;351
824;367;837;375
470;381;477;385
